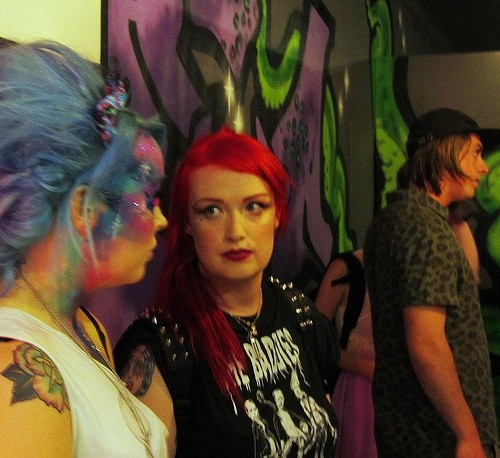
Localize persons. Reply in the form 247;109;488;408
363;108;500;458
0;41;169;458
317;248;380;458
112;127;338;458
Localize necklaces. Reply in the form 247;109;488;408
12;266;153;457
216;285;263;335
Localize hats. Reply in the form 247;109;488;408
406;108;500;158
